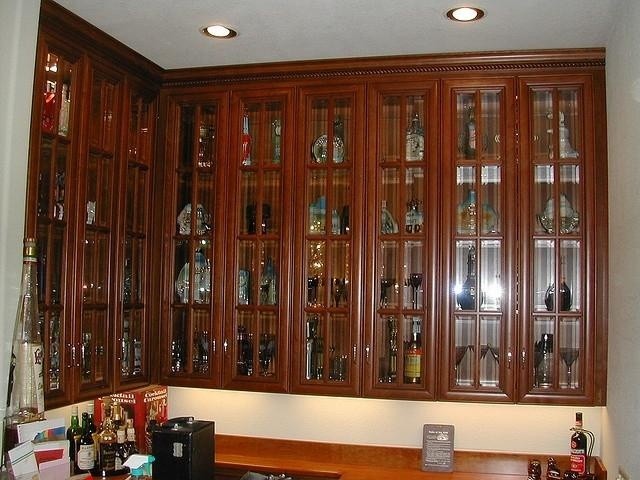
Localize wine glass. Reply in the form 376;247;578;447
308;277;345;307
533;330;580;392
380;273;422;309
455;344;500;387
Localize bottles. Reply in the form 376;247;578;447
67;401;137;473
241;114;253;165
3;238;45;430
544;255;572;311
466;190;477;236
405;112;425;161
404;317;422;384
333;114;344;139
570;412;587;477
466;107;477;159
406;189;423;233
120;327;135;376
456;244;485;310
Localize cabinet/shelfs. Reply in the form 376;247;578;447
165;47;606;411
25;0;165;409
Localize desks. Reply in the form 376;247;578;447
214;433;607;479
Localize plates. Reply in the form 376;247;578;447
312;134;346;163
455;201;497;235
